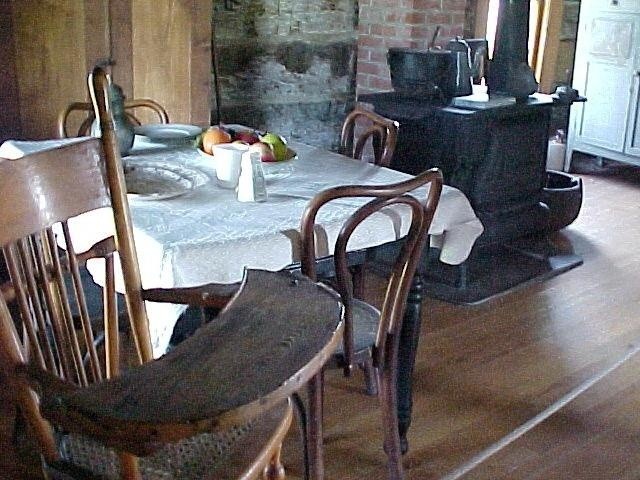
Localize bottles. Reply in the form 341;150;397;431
89;84;134;158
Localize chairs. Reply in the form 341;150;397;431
199;168;443;477
2;66;346;479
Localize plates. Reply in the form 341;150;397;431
119;157;212;202
134;122;204;146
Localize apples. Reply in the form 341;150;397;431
203;126;231;155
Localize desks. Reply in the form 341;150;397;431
356;89;587;307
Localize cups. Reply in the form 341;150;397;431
212;141;249;190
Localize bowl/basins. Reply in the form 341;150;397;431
196;123;300;175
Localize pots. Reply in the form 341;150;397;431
385;44;453;107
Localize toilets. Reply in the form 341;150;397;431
542;169;584;256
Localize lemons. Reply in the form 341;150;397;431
260;133;288;161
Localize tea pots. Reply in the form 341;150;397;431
445;35;486;96
